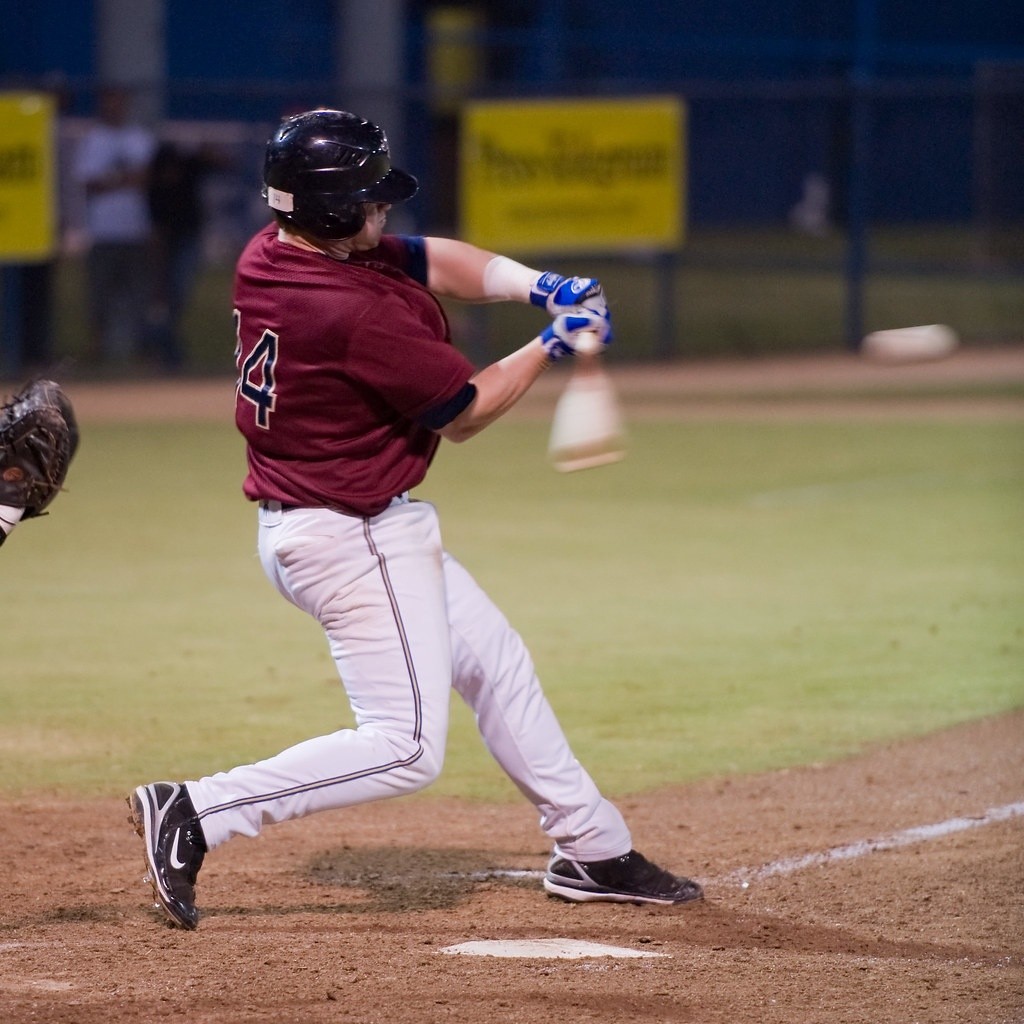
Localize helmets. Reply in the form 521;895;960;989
254;108;421;244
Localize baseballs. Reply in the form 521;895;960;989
857;323;958;369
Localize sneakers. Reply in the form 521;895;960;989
125;780;208;932
543;846;704;910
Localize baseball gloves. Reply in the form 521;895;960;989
0;377;82;549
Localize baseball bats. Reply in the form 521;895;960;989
549;329;631;474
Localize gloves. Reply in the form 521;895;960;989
529;269;610;320
537;310;612;363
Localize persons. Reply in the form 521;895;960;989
21;82;206;377
126;107;704;929
0;380;80;546
